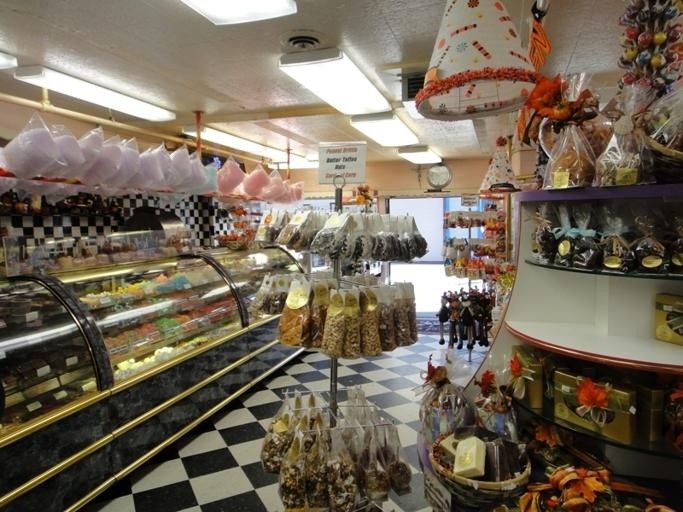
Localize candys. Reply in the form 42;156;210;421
617;0;683;91
0;128;303;204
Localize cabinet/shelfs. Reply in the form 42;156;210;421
0;242;308;512
459;182;683;510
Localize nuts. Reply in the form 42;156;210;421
254;222;428;359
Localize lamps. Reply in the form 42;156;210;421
13;66;177;122
279;49;393;116
182;124;307;166
398;145;442;164
182;0;297;27
350;112;419;148
0;52;17;72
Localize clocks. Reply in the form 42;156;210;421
427;164;452;188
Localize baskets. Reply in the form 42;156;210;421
429;435;531;512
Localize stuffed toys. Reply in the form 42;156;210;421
435;203;513;350
